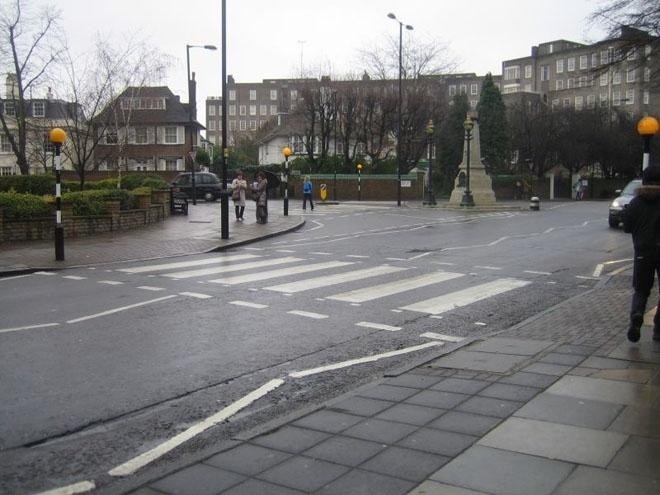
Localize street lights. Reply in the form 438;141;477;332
357;164;363;201
186;46;217;205
49;127;67;261
422;119;438;206
459;115;475;207
637;115;659;178
387;14;414;207
282;147;291;216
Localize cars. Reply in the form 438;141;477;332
607;178;642;229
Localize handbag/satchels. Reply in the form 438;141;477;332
232;191;240;200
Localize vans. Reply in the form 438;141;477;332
170;171;223;202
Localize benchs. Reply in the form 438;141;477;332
170;187;188;215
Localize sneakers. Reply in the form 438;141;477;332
627;312;660;342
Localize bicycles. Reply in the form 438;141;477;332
575;183;584;201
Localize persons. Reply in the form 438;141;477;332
230;172;247;223
302;175;314;211
515;182;523;200
256;172;267;224
622;164;659;342
572;178;583;200
250;173;268;223
522;179;528;200
